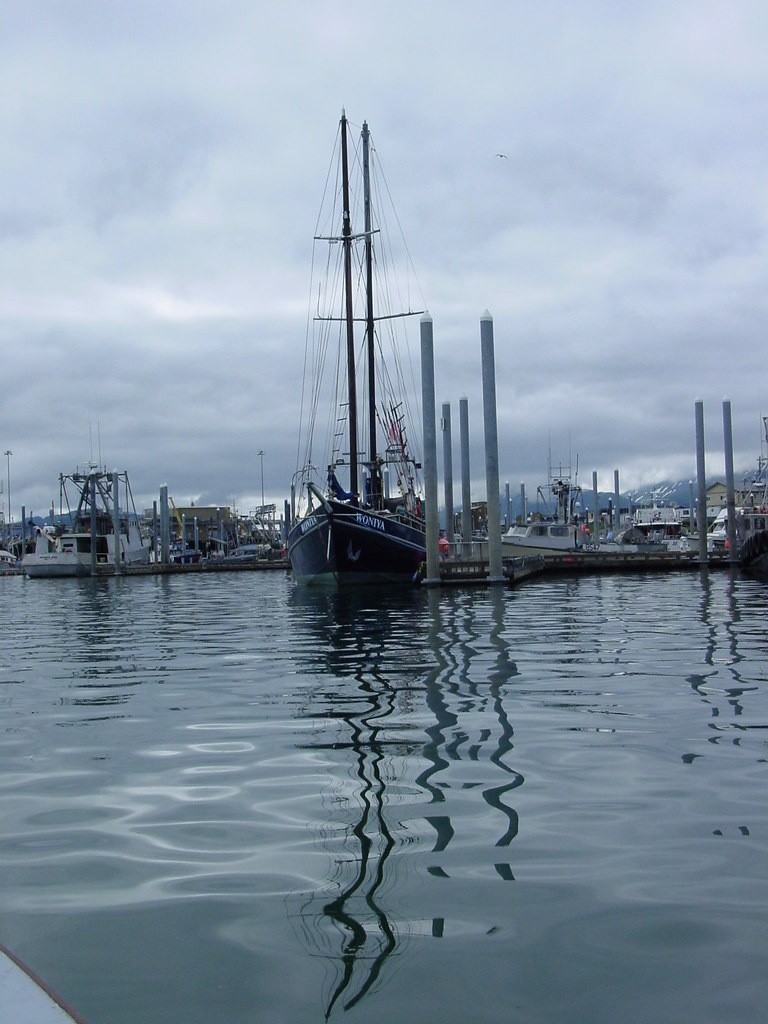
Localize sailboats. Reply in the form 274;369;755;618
0;416;768;578
280;104;440;599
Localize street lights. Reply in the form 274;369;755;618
257;449;265;545
3;449;14;541
